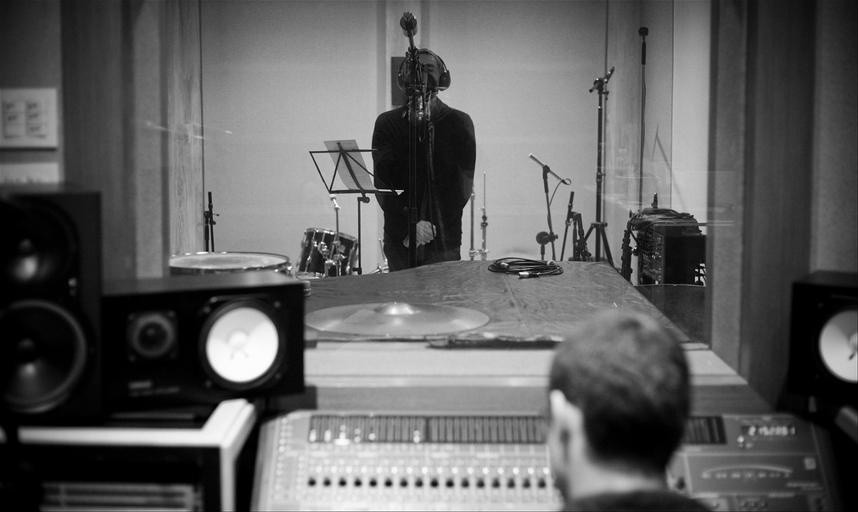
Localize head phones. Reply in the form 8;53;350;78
397;49;450;91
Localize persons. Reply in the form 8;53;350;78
540;310;719;512
371;48;476;271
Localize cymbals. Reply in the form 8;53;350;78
304;301;490;337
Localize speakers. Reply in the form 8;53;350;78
103;269;305;413
0;182;115;427
788;270;858;443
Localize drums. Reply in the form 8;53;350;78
295;228;358;279
167;251;290;275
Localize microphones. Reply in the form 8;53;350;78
604;67;615;83
329;194;339;209
529;154;567;184
639;27;648;43
400;12;418;37
